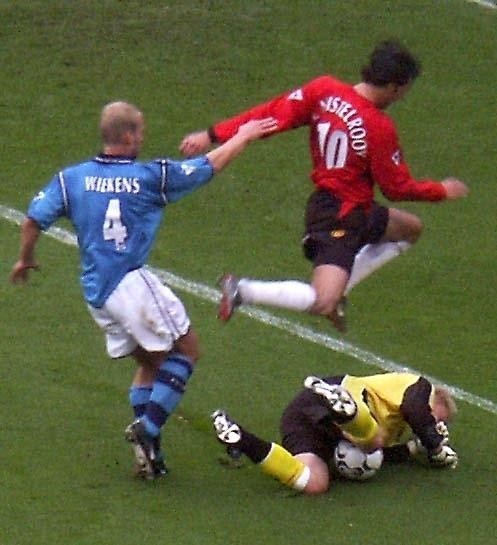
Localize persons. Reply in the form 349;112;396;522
210;371;459;497
10;100;279;482
177;39;473;337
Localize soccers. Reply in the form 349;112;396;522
335;440;384;479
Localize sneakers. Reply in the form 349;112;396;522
326;296;348;334
124;419;169;484
220;272;242;321
213;411;243;447
303;374;357;420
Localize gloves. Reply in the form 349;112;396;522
428;446;459;471
437;419;451;446
